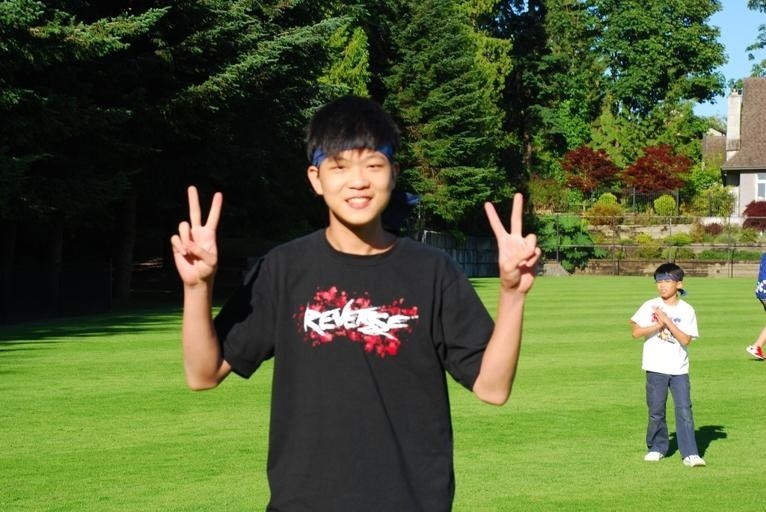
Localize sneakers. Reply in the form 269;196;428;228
644;452;664;461
683;455;706;467
746;345;766;359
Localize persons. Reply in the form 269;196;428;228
745;253;766;360
167;96;544;512
628;263;706;469
